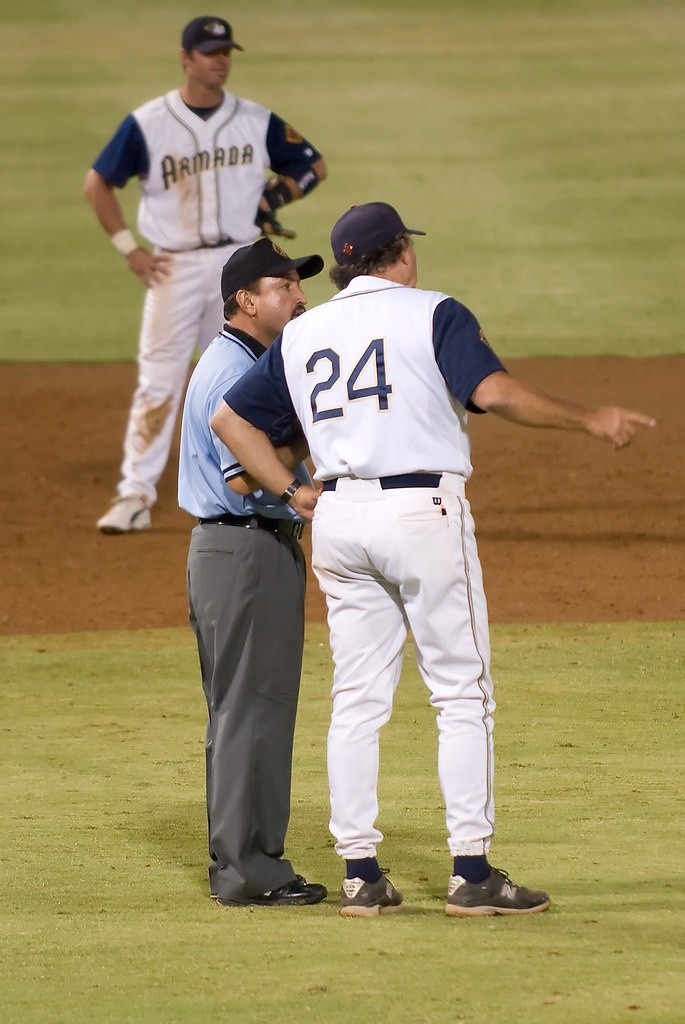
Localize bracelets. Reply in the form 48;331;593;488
263;182;292;210
109;229;137;255
279;479;304;505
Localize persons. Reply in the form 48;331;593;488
175;237;326;908
210;201;657;918
84;17;326;533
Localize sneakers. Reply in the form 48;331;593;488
445;868;550;919
96;494;152;531
219;872;327;906
340;867;404;915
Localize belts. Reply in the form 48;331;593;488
159;239;237;252
198;514;303;537
320;470;443;491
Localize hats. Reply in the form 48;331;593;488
220;238;325;299
331;201;427;263
181;16;248;54
